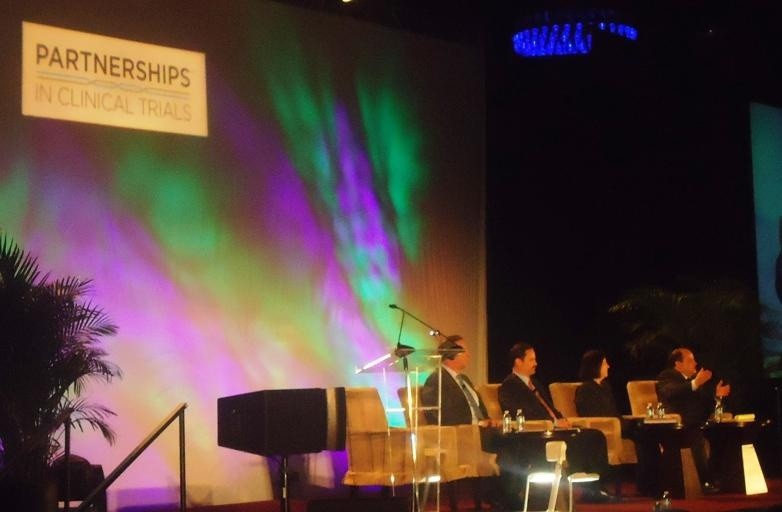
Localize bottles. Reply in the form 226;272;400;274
656;402;665;419
661;491;673;512
714;401;723;422
646;402;654;419
502;410;512;434
516;408;526;432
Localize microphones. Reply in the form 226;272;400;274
389;304;397;309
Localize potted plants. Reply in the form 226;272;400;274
0;233;116;512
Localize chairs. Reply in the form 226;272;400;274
341;382;681;511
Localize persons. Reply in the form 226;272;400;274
423;333;548;511
497;340;630;502
654;346;732;492
573;347;684;499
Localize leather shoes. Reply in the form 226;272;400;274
702;482;720;493
582;488;618;502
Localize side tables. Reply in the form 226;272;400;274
699;420;771;495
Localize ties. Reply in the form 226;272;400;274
527;380;558;420
456;374;484;420
